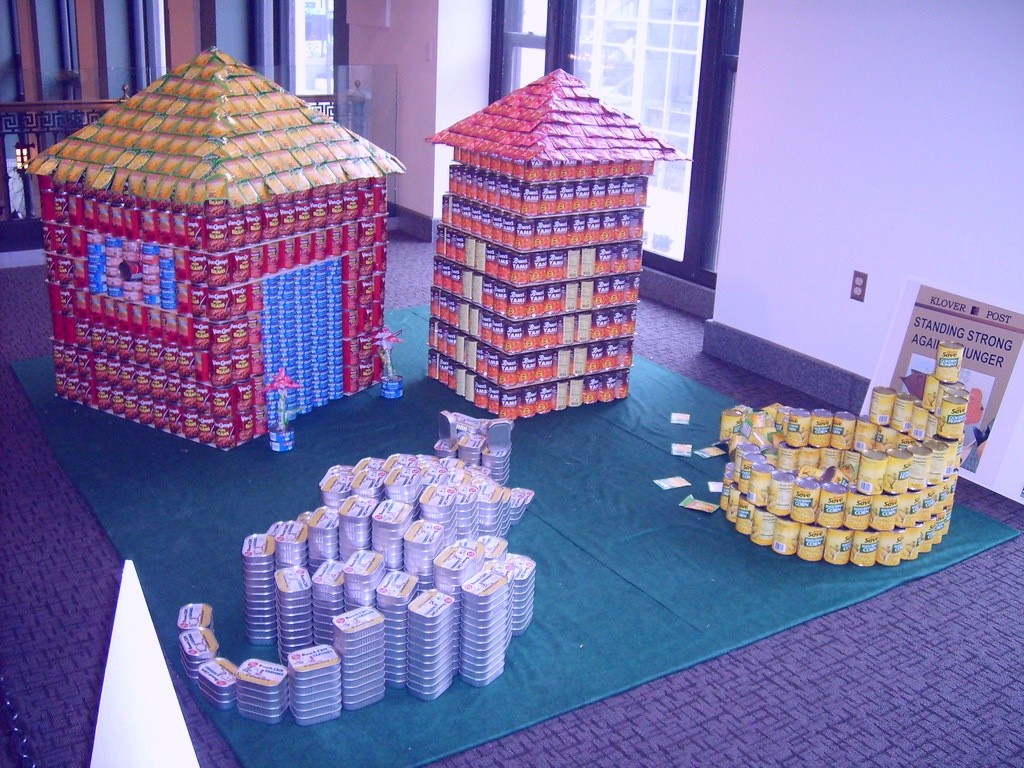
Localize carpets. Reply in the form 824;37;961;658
10;307;1021;768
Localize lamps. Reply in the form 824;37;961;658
14;141;31;179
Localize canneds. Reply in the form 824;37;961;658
37;173;404;452
176;415;537;726
720;342;971;567
426;145;653;422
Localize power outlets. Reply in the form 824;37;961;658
850;270;868;302
